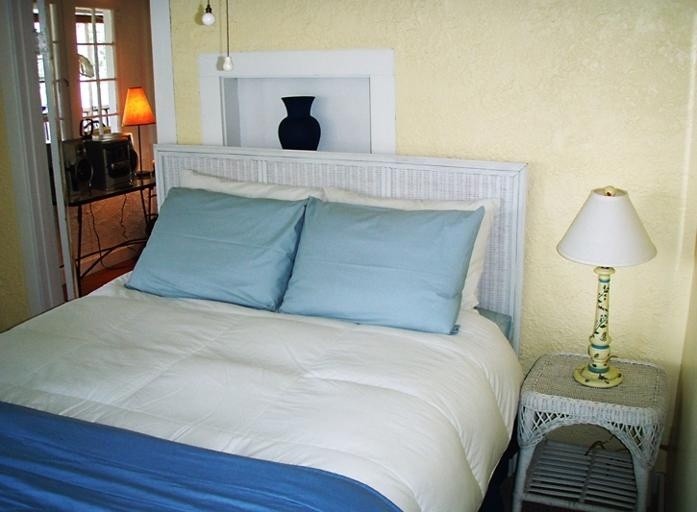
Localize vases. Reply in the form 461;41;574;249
274;96;321;150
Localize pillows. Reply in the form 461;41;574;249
322;185;497;316
123;185;309;314
180;169;322;201
277;194;487;339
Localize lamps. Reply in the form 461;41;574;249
120;87;156;176
201;1;233;73
555;187;658;389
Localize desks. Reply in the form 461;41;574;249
53;171;154;297
511;355;664;511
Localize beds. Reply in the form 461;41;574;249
0;146;526;512
